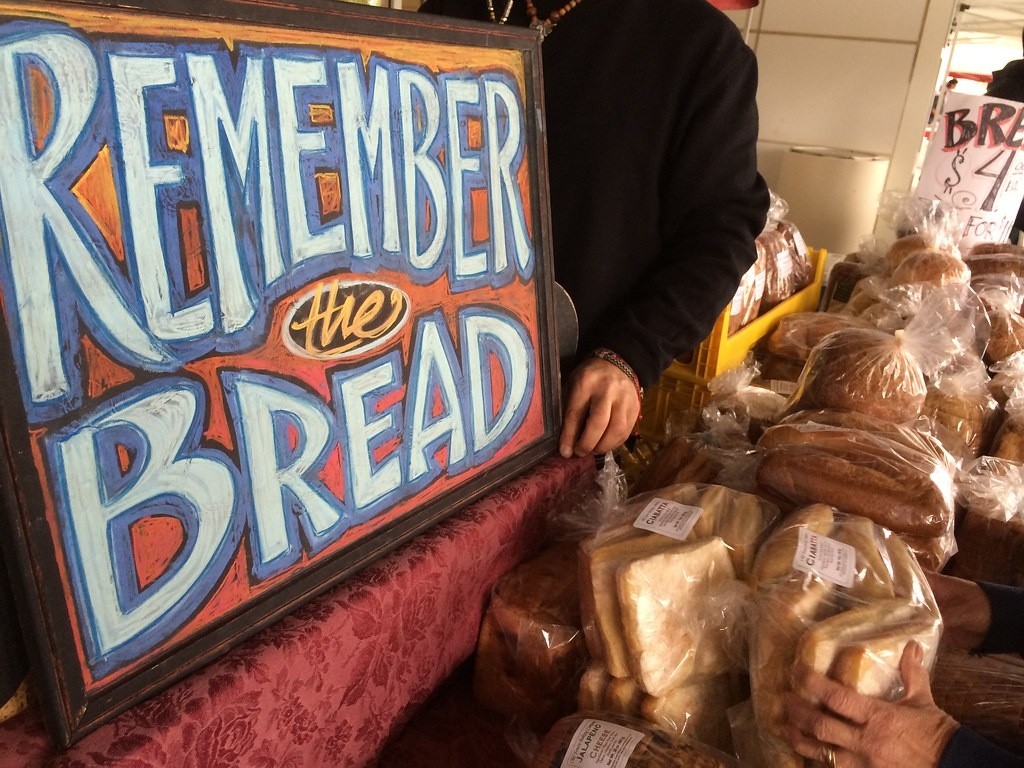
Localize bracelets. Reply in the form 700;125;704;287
595;347;644;436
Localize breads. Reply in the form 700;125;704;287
472;221;1024;768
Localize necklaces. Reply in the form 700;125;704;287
487;0;584;43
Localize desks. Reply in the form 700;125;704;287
0;451;599;768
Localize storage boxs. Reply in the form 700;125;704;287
637;245;829;437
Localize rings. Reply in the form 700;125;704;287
824;749;837;768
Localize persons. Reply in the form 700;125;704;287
783;565;1024;768
412;0;769;462
980;27;1024;233
930;79;959;124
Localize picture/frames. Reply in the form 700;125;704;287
0;0;571;750
